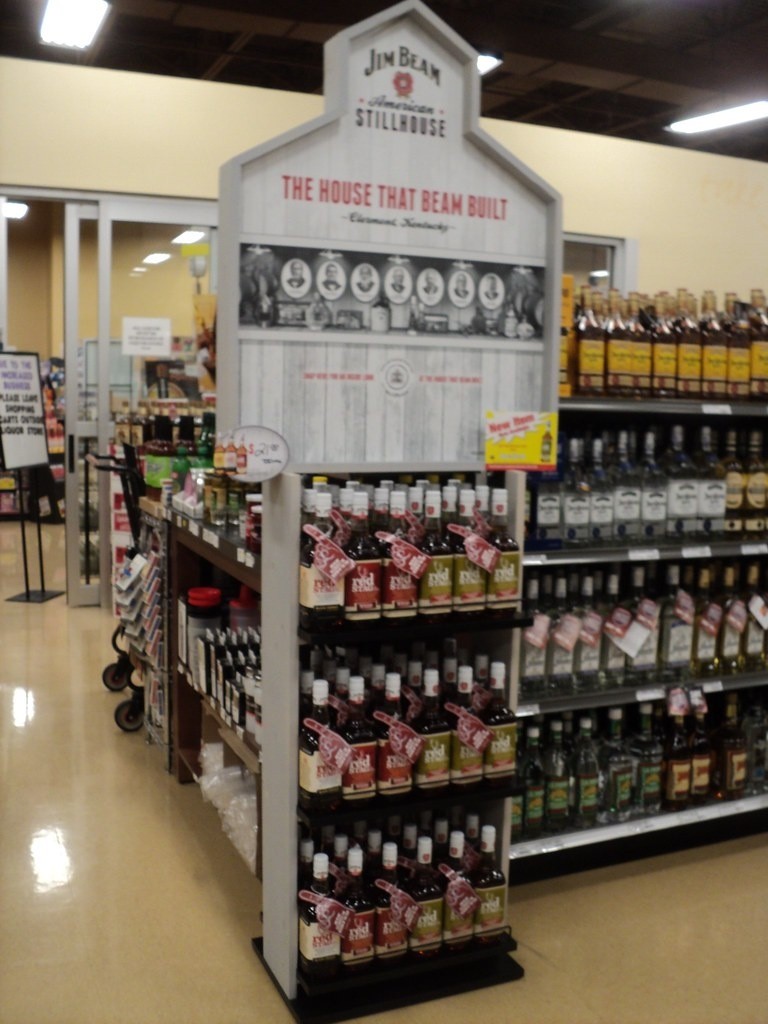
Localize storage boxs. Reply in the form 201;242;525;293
139;551;161;657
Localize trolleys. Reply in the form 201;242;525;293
90;450;149;730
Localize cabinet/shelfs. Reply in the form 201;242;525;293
162;394;768;1024
0;487;32;521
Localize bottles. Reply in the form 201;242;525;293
117;277;767;977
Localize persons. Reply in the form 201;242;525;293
485;275;498;299
423;272;438;294
323;264;341;290
454;274;468;296
287;261;305;288
392;268;404;292
357;266;374;291
